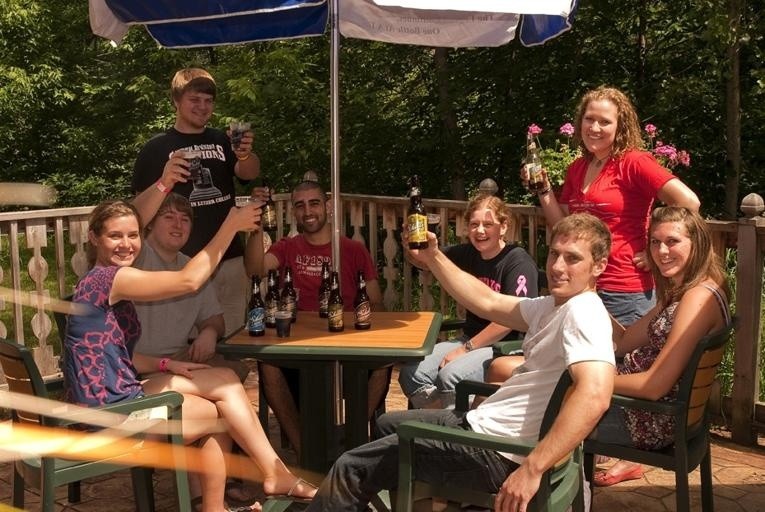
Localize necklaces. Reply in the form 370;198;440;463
483;245;502;259
593;155;609;168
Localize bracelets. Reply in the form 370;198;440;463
236;155;249;161
159;357;170;372
465;339;474;351
154;177;172;193
540;182;553;196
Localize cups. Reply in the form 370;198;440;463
180;150;201;181
230;120;249;151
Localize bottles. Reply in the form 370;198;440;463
354;272;372;330
262;186;278;232
407;174;429;250
319;262;331;318
246;265;298;337
526;141;546;193
327;270;345;332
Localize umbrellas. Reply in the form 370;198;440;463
88;0;576;427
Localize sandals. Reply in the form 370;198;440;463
590;459;648;486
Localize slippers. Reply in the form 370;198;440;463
185;493;207;511
279;445;305;466
320;439;345;472
262;476;327;501
222;478;257;507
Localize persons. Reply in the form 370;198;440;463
470;205;732;451
398;193;539;409
302;212;617;511
132;67;261;338
131;152;255;511
62;200;320;511
244;180;389;452
520;86;702;488
